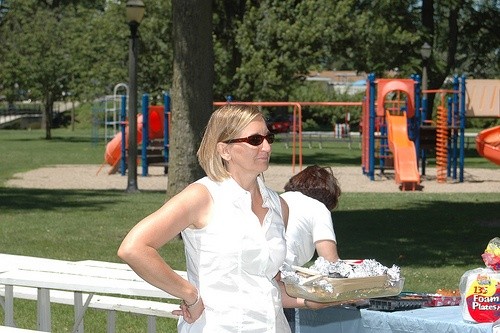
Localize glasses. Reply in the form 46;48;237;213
222;132;274;146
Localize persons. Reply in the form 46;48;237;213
276;165;341;266
117;103;367;333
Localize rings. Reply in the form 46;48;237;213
340;303;343;306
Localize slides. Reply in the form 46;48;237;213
385;109;421;189
475;122;500;165
105;106;165;172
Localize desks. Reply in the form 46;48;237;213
285;296;500;333
0;254;362;332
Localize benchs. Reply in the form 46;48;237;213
0;286;193;332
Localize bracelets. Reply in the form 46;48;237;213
304;298;311;309
183;287;200;306
